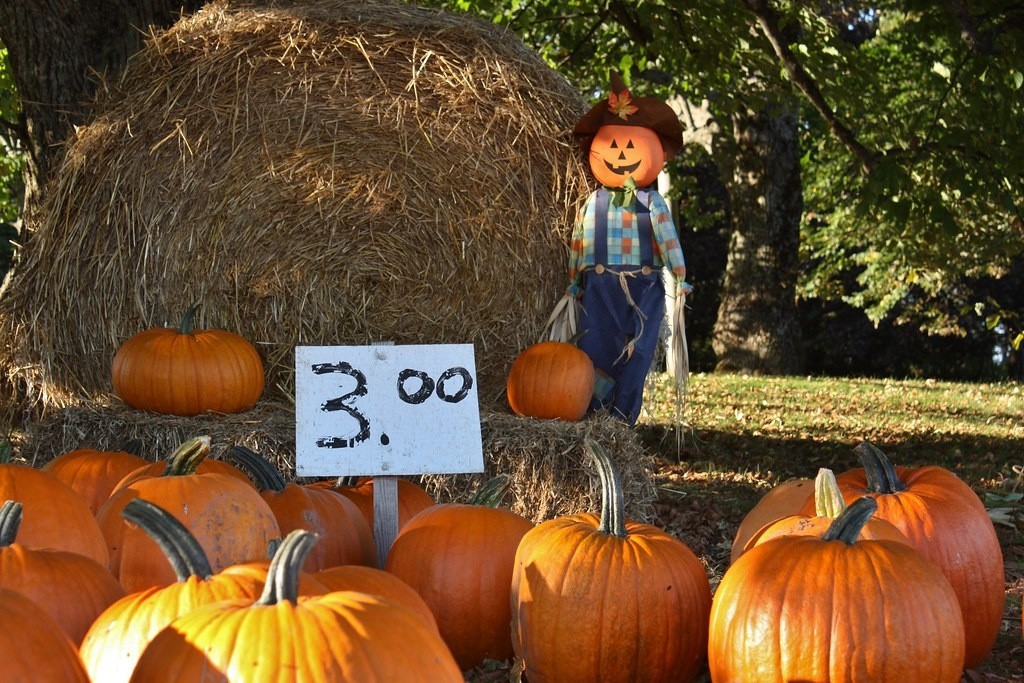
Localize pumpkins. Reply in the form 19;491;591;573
0;437;1004;683
506;328;595;420
112;300;264;415
